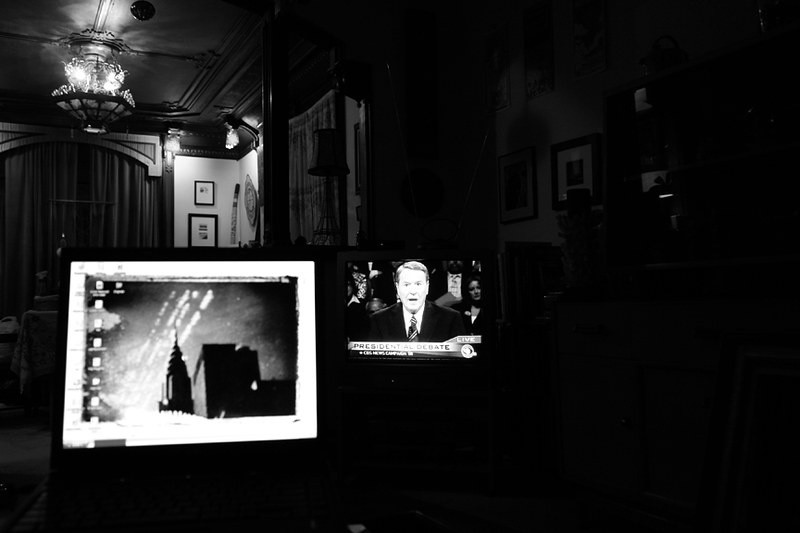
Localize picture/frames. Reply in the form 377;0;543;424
497;146;538;226
480;0;608;118
194;180;214;205
354;123;362;195
552;133;603;212
188;213;218;247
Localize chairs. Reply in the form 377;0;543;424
685;325;800;533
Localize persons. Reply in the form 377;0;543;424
347;260;481;342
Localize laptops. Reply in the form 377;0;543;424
0;244;336;533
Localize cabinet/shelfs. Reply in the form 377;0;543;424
335;387;507;498
556;21;800;526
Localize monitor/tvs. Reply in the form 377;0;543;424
334;247;498;368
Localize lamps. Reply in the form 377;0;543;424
51;40;136;134
307;129;350;247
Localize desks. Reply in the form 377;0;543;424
9;310;59;433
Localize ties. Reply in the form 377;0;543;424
409;316;419;344
